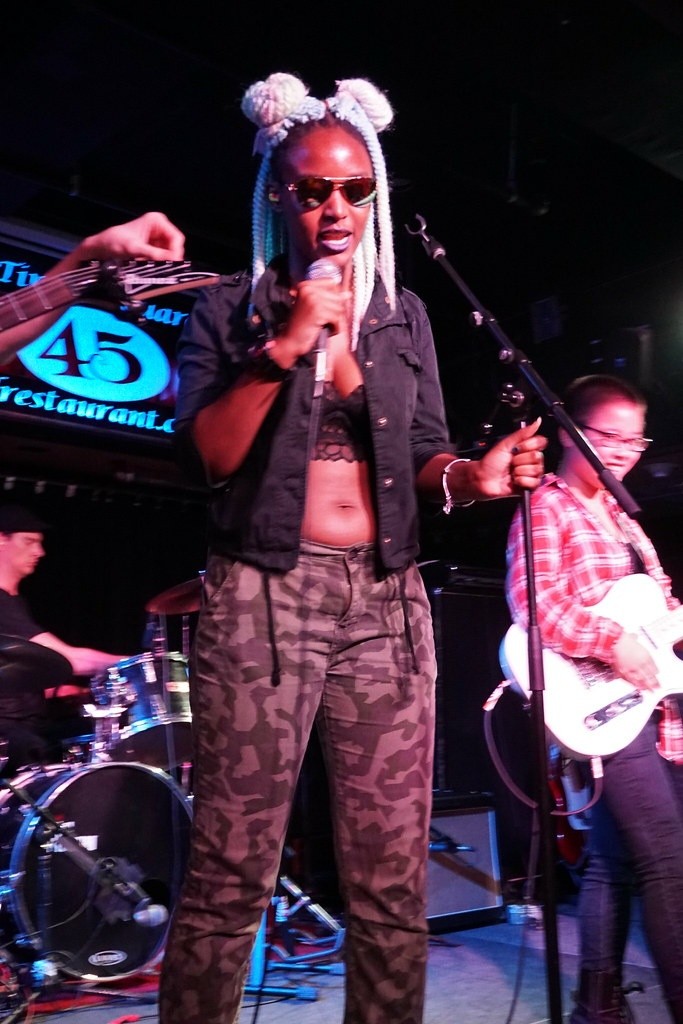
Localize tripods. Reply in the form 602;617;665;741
0;777;158;1024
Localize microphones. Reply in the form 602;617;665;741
304;259;344;398
426;841;476;854
112;873;168;927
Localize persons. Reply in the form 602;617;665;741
159;73;547;1024
0;212;185;367
504;376;683;1024
0;511;129;962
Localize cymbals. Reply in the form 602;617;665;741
142;576;199;616
0;633;75;694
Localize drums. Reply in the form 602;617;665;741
58;734;94;764
85;650;195;772
0;759;193;983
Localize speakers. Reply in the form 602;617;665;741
423;790;506;934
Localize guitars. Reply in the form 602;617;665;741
496;572;683;761
0;256;245;333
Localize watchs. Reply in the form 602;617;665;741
246;339;295;382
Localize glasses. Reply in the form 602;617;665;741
281;175;377;208
577;423;653;452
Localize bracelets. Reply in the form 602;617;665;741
443;458;476;513
